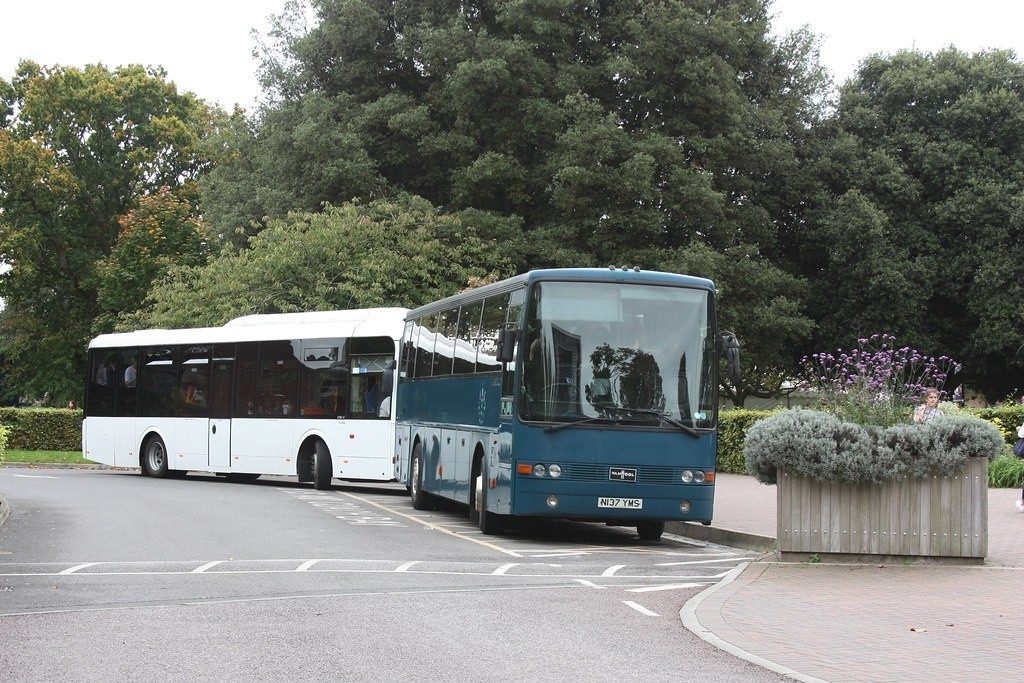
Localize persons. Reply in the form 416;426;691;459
913;388;942;424
95;352;137;388
1015;422;1023;512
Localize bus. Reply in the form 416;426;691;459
82;306;415;490
392;264;743;540
